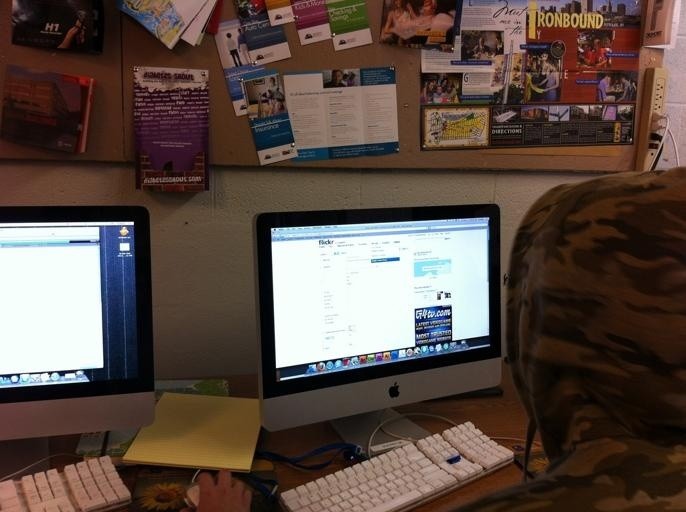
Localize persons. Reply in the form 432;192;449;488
225;32;243;67
348;71;360;86
12;0;47;28
68;10;98;50
445;163;686;510
246;3;265;23
255;77;285;115
237;28;252;65
323;69;347;88
184;469;257;511
377;1;637;105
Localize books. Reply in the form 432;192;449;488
75;376;268;473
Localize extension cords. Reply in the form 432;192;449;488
636;61;670;171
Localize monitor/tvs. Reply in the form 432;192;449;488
0;204;156;474
252;202;503;456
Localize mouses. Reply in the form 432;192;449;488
184;478;202;510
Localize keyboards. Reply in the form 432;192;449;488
1;449;133;511
278;418;514;511
